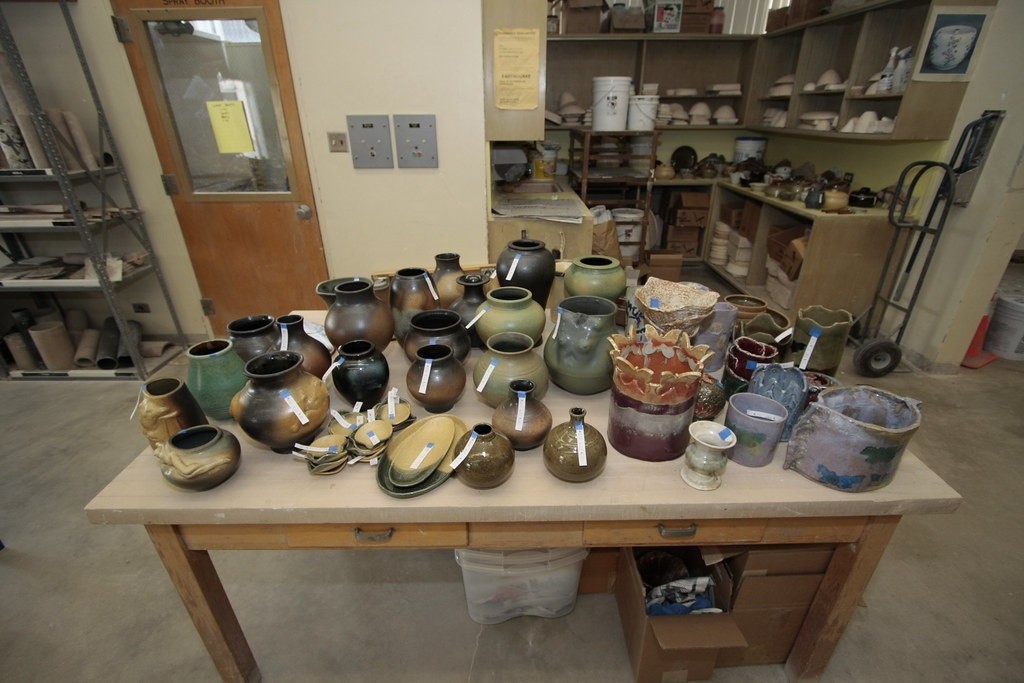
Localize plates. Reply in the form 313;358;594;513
375;413;469;499
388;416;455;487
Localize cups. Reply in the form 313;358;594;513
929;23;978;72
729;172;741;184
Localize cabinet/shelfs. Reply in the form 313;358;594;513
641;175;729;263
746;0;998;140
704;180;915;337
0;0;190;382
544;33;760;129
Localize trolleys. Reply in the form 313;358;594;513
846;160;957;377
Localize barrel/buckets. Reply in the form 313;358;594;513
627;95;660;132
734;136;767;164
983;294;1024;361
527;148;556;182
591;75;632;132
610;208;645;258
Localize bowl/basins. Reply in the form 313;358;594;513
557;69;883;212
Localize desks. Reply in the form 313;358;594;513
83;309;965;682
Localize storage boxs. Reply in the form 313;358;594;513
766;214;812;280
720;198;760;243
454;544;834;683
765;0;831;32
547;0;714;34
635;192;710;281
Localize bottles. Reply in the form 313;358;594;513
136;240;924;497
801;188;811;201
791;175;802;193
804;191;825;208
708;7;725;34
546;15;559;36
